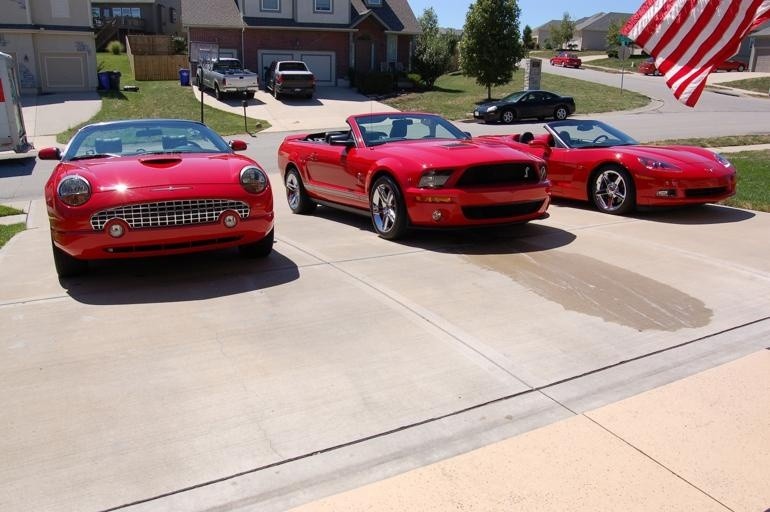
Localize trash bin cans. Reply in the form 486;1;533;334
98;71;121;91
179;69;190;86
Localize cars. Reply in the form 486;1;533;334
641;56;664;76
473;89;576;124
264;59;316;99
550;52;582;68
710;57;748;73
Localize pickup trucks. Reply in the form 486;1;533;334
196;57;259;100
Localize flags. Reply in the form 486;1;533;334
618;0;770;107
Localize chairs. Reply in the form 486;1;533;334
161;135;188;150
325;131;349;144
94;136;122;153
555;131;570;148
518;132;536;144
